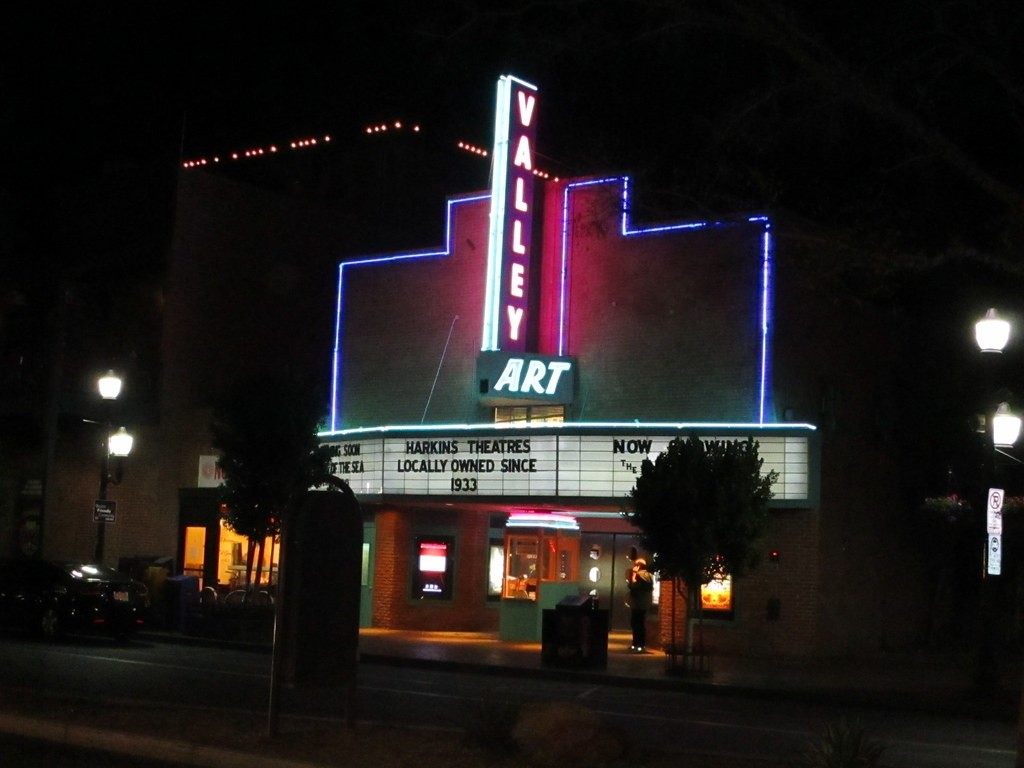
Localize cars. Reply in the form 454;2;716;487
0;548;149;644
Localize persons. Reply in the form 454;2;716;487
625;558;655;655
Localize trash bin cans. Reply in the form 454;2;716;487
541;596;611;670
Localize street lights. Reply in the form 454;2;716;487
973;306;1024;664
90;365;138;568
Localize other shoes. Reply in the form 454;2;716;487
624;645;646;654
